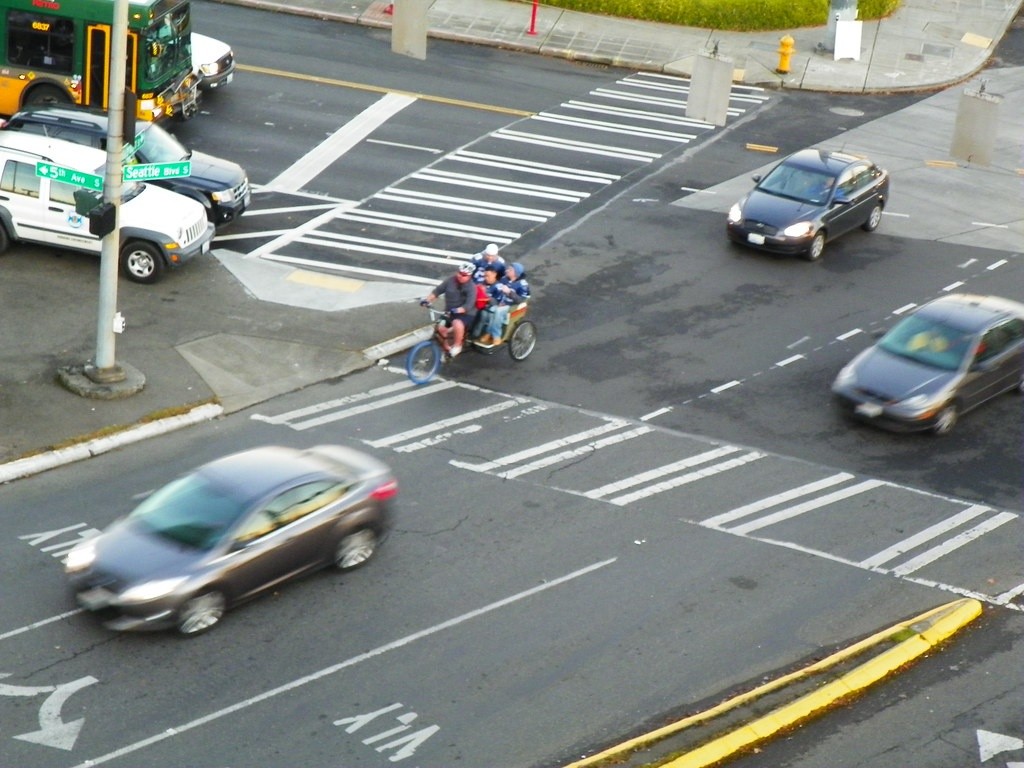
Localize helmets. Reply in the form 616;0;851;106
459;261;476;276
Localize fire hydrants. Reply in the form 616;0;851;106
775;34;797;74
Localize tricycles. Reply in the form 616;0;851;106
407;300;538;385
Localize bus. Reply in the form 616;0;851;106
0;0;203;130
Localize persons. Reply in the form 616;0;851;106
421;262;479;359
469;244;529;346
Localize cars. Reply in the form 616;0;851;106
62;442;397;637
724;147;890;259
189;31;236;91
830;292;1024;437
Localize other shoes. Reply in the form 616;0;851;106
441;350;450;363
493;339;502;345
449;346;462;357
480;334;490;342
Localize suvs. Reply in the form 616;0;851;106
0;130;218;285
0;103;252;230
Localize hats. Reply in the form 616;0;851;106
485;244;498;255
511;262;524;277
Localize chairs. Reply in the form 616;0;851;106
782;168;818;196
